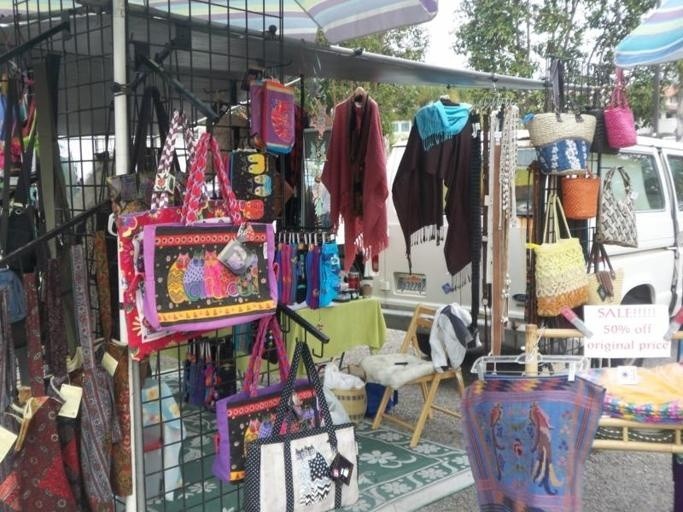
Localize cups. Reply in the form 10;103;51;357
348;272;360;293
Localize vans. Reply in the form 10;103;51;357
367;132;683;355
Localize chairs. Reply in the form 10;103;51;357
371;305;467;448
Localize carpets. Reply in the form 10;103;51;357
138;359;476;512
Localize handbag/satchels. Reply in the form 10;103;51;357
525;95;596;177
104;84;187;217
275;231;341;309
245;341;358;512
603;86;637;149
213;316;320;482
559;171;601;220
115;113;265;361
249;67;277;155
7;52;63;276
532;194;590;317
141;134;278;332
0;231;127;512
597;165;639;249
581;87;620;155
262;63;296;154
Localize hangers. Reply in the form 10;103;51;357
353;82;367;101
469;326;590;381
438;85;462;110
